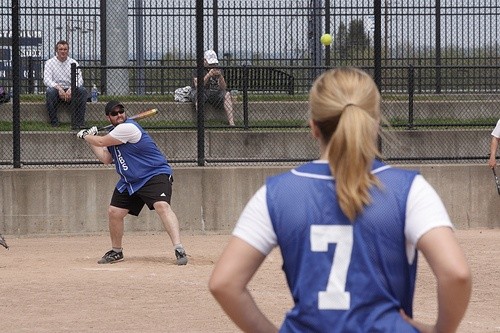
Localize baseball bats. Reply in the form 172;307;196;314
76;107;159;138
487;153;500;195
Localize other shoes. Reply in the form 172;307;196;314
72;126;89;130
51;121;59;128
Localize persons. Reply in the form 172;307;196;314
208;67;472;333
77;100;187;266
488;119;500;168
44;41;88;129
189;48;234;127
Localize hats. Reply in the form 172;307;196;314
203;49;218;64
105;100;124;114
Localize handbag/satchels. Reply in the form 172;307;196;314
174;86;193;102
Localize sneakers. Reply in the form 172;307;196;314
98;249;124;263
175;245;188;264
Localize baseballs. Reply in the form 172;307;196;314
319;33;333;45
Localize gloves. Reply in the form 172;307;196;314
87;126;98;136
77;129;87;138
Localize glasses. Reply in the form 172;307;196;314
108;109;124;116
